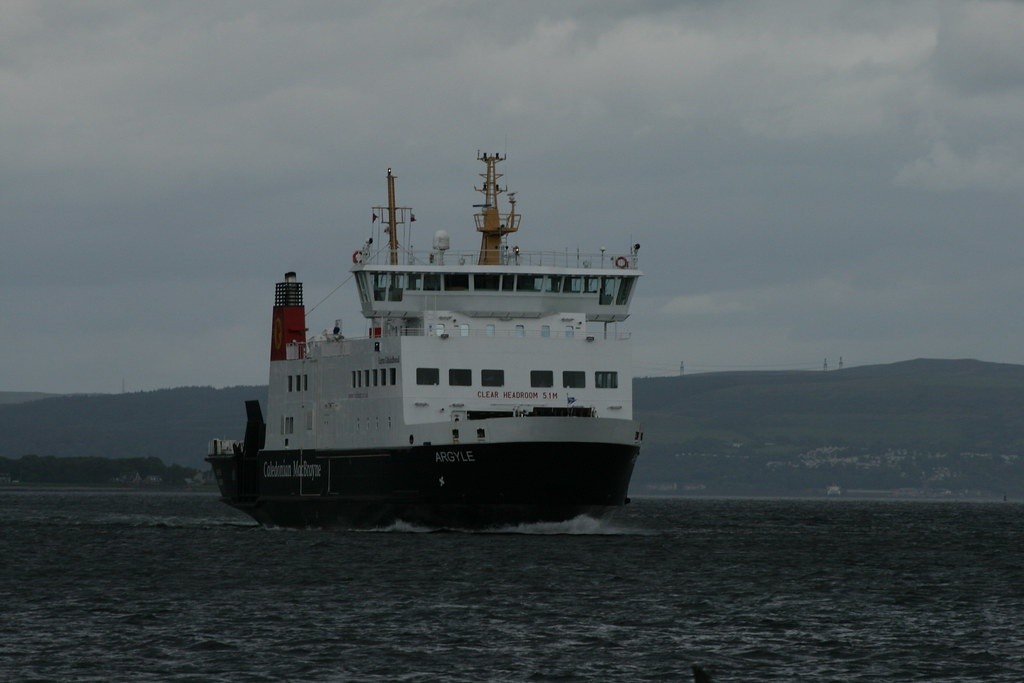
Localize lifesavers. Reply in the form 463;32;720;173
615;256;628;269
352;250;364;264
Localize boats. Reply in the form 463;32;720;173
205;148;647;528
